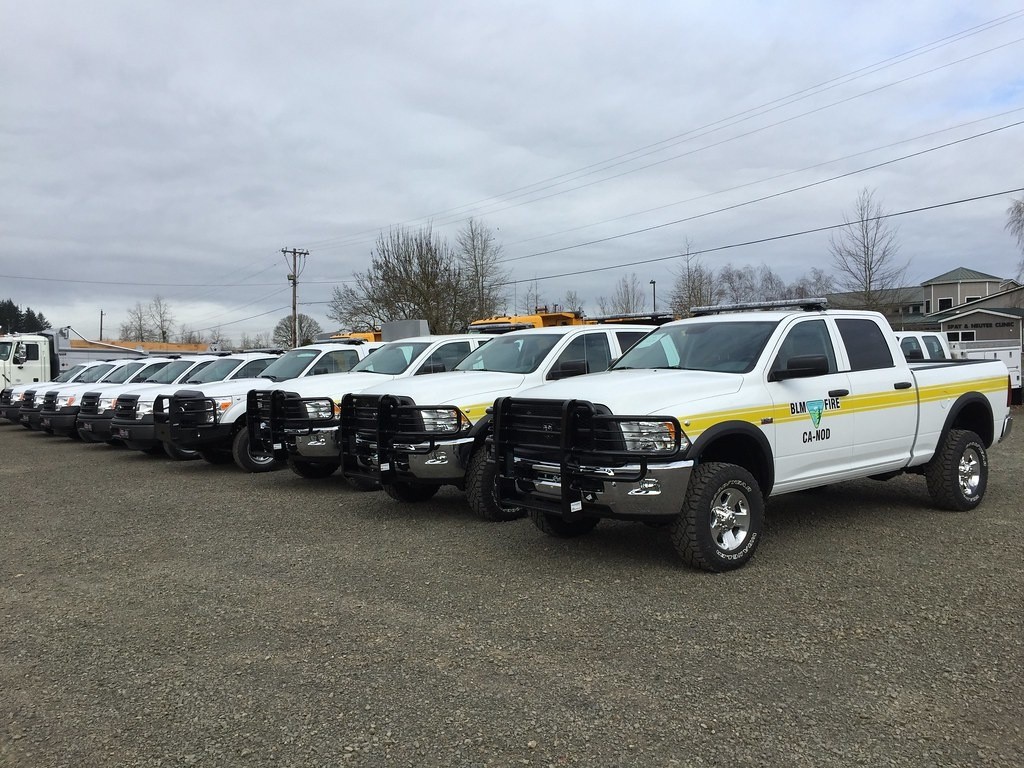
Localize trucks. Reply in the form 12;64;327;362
0;325;181;390
470;311;676;335
329;332;381;371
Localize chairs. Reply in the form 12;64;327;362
787;354;829;376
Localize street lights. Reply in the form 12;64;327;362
650;281;657;310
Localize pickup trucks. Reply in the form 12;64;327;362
247;334;504;488
892;332;1021;404
0;353;313;472
338;325;680;518
488;311;1013;572
